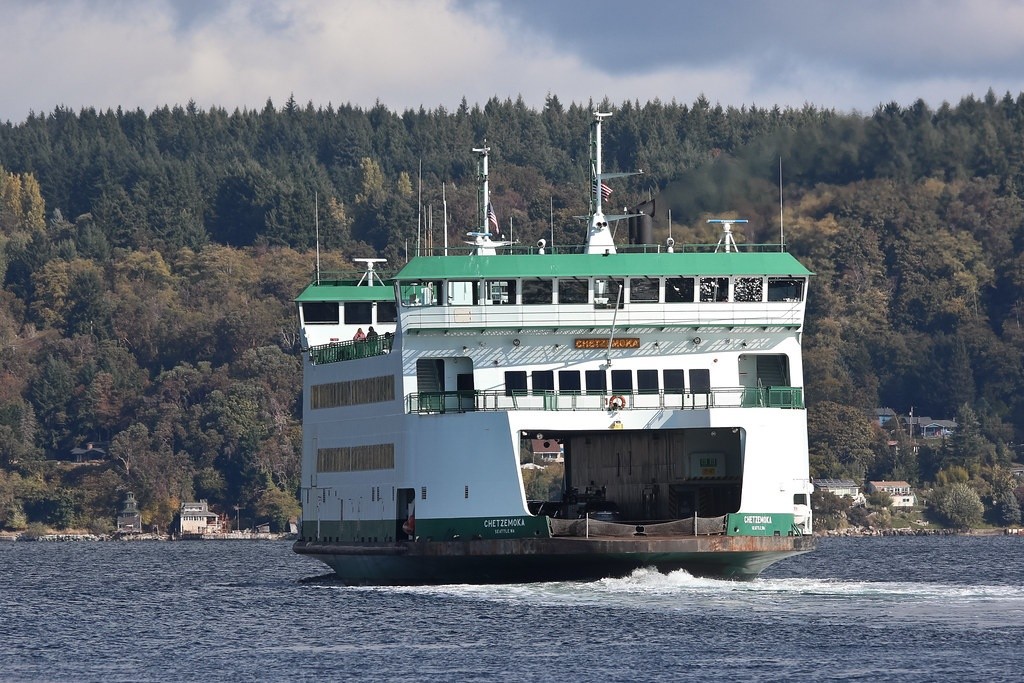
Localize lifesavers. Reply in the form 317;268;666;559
609;395;626;410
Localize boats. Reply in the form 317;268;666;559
287;109;821;581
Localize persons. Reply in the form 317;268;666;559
366;326;378;355
353;328;366;357
385;332;392;353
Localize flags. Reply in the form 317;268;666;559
488;202;499;234
593;180;613;202
